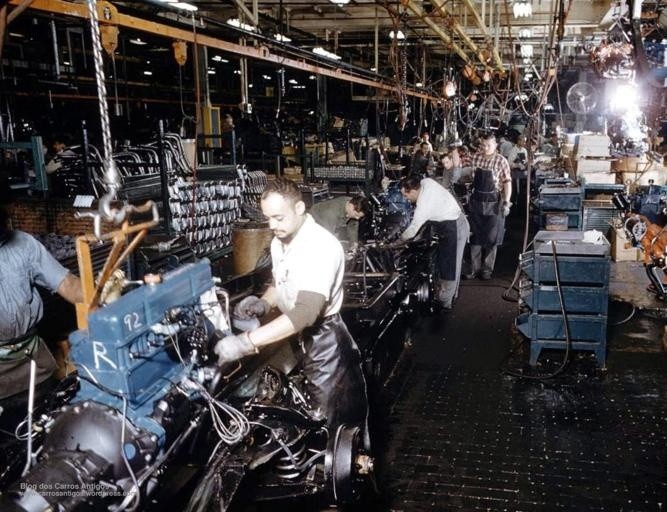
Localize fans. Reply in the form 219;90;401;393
566;81;599;134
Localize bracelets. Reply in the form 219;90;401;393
503;200;512;210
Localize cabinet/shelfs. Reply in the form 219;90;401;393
518;144;611;372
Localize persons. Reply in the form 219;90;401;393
307;194;372;255
457;145;472;168
213;177;376;462
1;158;124;432
499;129;520;159
410;142;438;178
414;132;435;162
507;134;530;202
389;176;471;315
550;133;557;147
464;133;512;279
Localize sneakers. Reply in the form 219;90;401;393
436;271;492;315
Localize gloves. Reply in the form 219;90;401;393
213;329;259;368
500;201;513;217
97;268;125;306
232;294;271;321
